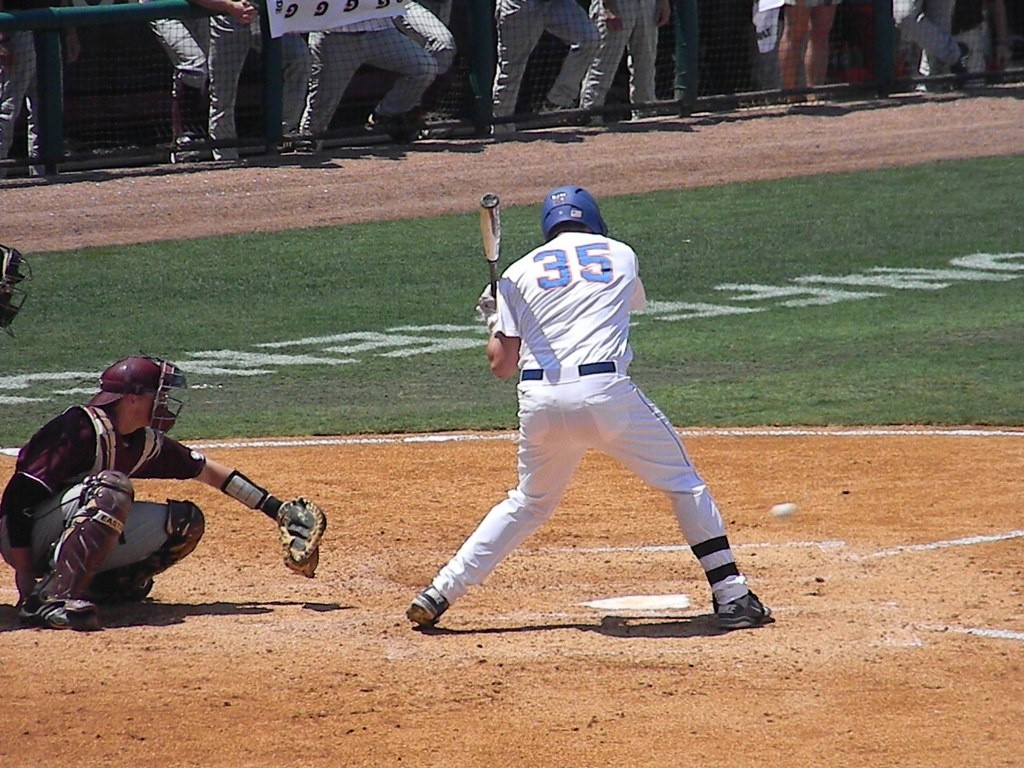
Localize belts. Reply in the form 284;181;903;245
521;361;616;381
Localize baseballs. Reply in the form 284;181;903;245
767;502;798;517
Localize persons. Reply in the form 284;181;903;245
0;349;327;629
0;0;1024;178
407;184;772;629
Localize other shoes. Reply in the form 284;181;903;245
911;41;972;93
533;98;577;129
365;111;404;134
490;116;520;138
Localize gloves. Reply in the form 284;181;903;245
477;281;499;314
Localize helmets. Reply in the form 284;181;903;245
85;356;167;409
541;185;609;241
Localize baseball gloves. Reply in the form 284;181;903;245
277;497;327;578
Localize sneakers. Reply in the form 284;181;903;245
713;575;772;630
19;594;95;627
405;584;450;629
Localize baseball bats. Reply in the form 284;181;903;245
479;192;502;298
